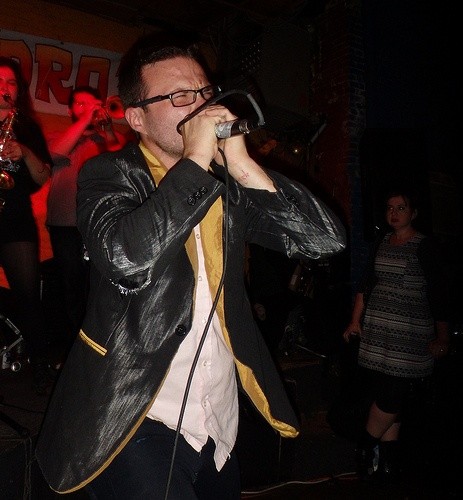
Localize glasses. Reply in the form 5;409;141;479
132;83;222;107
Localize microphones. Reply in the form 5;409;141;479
215;121;262;140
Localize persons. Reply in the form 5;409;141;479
0;56;128;402
344;193;447;473
34;33;347;496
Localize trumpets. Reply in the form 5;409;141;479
88;95;124;125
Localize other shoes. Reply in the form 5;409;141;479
30;359;55;394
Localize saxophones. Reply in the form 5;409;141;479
0;94;19;191
